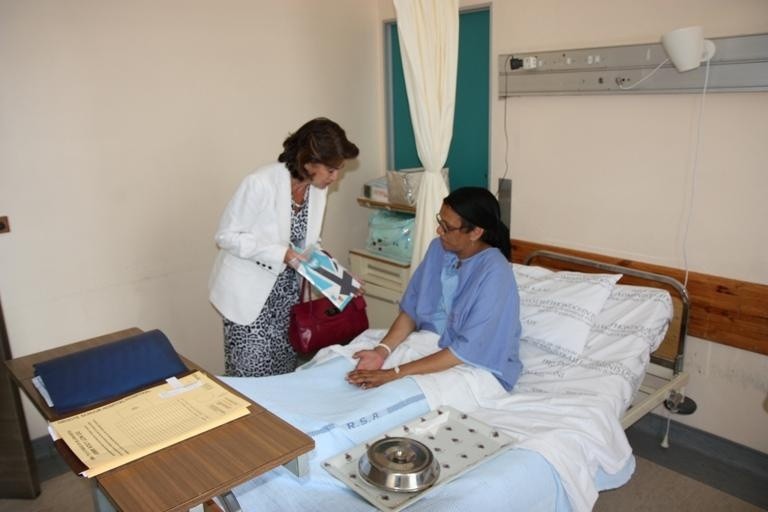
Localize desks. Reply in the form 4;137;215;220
4;326;316;511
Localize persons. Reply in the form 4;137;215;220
345;185;523;391
204;117;366;378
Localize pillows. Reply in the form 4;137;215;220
507;259;624;357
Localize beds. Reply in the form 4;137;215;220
89;247;689;512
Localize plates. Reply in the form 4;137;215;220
321;402;517;512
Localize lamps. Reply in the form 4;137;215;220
659;26;715;73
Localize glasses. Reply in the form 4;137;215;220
436;213;464;233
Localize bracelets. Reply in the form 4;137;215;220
377;344;391;353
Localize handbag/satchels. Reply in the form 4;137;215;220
291;290;368;354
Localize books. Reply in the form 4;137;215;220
47;370;252;479
287;242;363;313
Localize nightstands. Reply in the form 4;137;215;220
346;196;419;328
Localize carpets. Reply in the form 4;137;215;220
0;453;764;512
